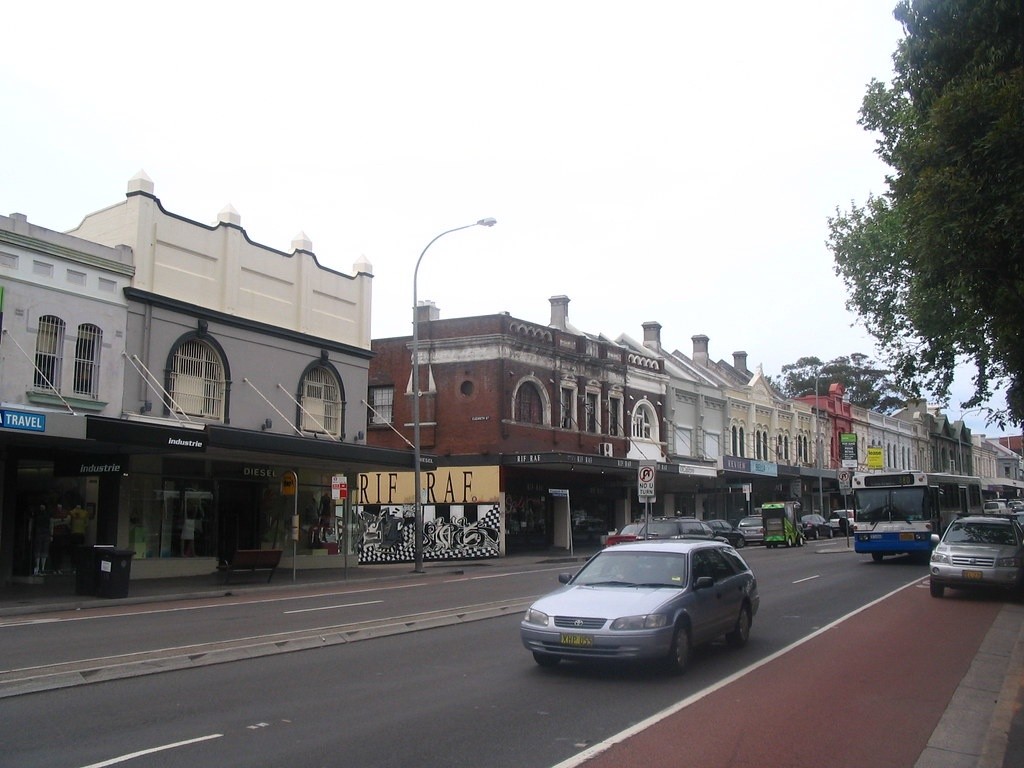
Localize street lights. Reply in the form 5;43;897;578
958;407;990;475
411;217;498;576
814;351;865;520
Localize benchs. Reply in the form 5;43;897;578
216;550;283;584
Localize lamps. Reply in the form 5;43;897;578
354;431;364;442
140;400;152;413
262;418;272;430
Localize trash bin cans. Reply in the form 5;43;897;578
96;548;137;599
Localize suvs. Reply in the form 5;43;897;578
605;515;731;549
830;509;865;535
928;510;1024;598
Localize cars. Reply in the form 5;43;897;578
983;498;1023;526
801;513;833;539
520;539;759;678
737;514;765;544
702;519;745;548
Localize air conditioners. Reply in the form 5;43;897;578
599;442;613;457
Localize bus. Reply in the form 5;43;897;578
850;469;984;567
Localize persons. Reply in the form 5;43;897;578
505;495;538;536
302;492;334;550
27;503;89;573
178;482;203;556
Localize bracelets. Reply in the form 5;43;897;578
49;535;53;537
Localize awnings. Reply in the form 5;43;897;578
503;452;981;485
0;403;436;475
